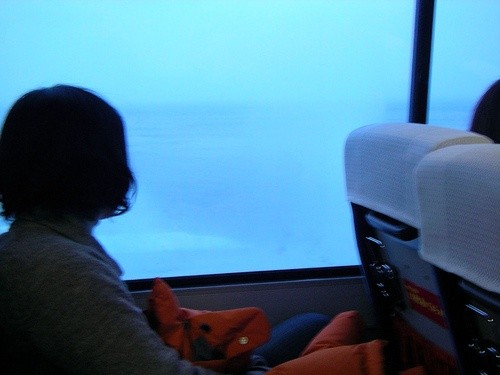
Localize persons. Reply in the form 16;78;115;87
0;83;334;375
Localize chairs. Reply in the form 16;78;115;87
343;121;494;374
416;145;499;375
